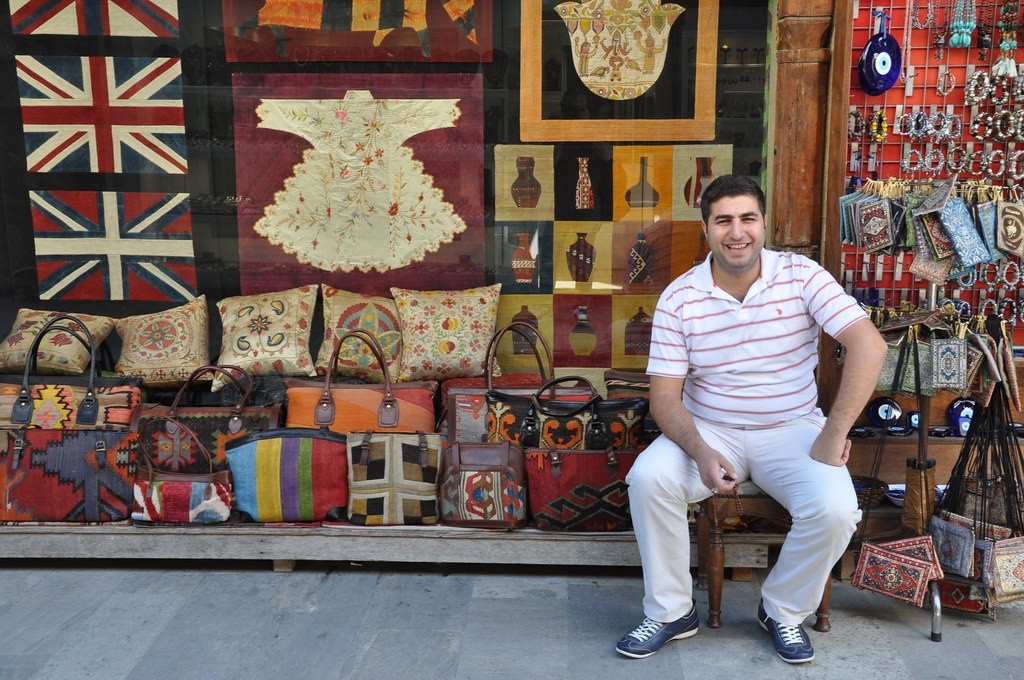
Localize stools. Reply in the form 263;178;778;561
698;480;832;633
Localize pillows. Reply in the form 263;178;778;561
0;307;118;374
111;294;214;388
388;283;504;384
210;283;320;392
314;282;403;385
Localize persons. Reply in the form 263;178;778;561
615;174;888;663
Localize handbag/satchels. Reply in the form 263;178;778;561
347;429;441;524
131;414;232;525
225;425;350;522
0;424;138;526
130;365;272;474
285;327;438;435
447;320;599;450
439;440;527;530
0;314;143;431
525;446;637;533
851;504;1024;623
518;376;664;453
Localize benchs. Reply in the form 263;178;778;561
0;296;324;406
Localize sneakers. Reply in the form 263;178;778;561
758;597;814;663
615;598;700;658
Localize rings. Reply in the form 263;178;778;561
711;487;718;493
